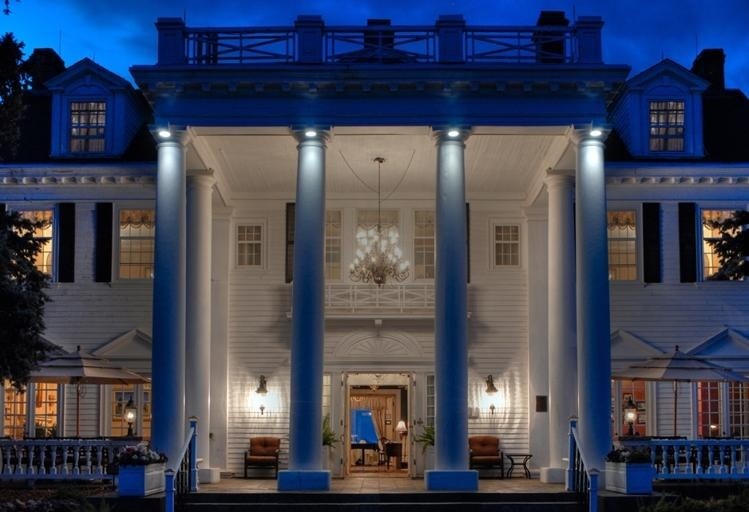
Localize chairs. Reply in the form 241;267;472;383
468;435;505;480
244;436;281;479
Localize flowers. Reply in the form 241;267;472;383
115;444;168;468
605;447;652;464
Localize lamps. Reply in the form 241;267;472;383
349;157;410;289
623;396;638;435
124;395;138;436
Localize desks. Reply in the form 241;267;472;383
506;453;534;480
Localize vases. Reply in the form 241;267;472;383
605;462;653;494
118;461;166;497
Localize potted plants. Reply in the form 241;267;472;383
322;412;338;469
414;422;435;469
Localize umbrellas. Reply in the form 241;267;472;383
11;343;151;438
610;344;748;436
